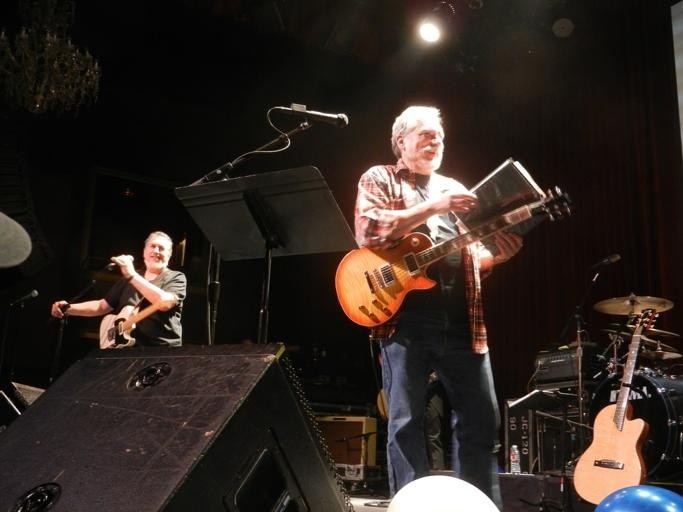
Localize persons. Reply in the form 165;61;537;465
50;228;187;346
353;104;523;508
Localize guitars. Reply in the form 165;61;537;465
98;293;179;350
334;185;572;328
573;307;660;505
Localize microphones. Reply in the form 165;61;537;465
273;105;350;130
104;255;134;273
9;289;39;307
599;253;621;269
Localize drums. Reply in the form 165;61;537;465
588;369;683;476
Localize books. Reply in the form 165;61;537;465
449;157;548;259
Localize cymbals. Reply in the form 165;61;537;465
592;293;674;318
638;348;682;361
627;321;680;339
600;327;677;351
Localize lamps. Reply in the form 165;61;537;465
411;1;465;48
0;1;104;119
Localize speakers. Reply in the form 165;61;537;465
0;341;354;512
432;469;540;511
